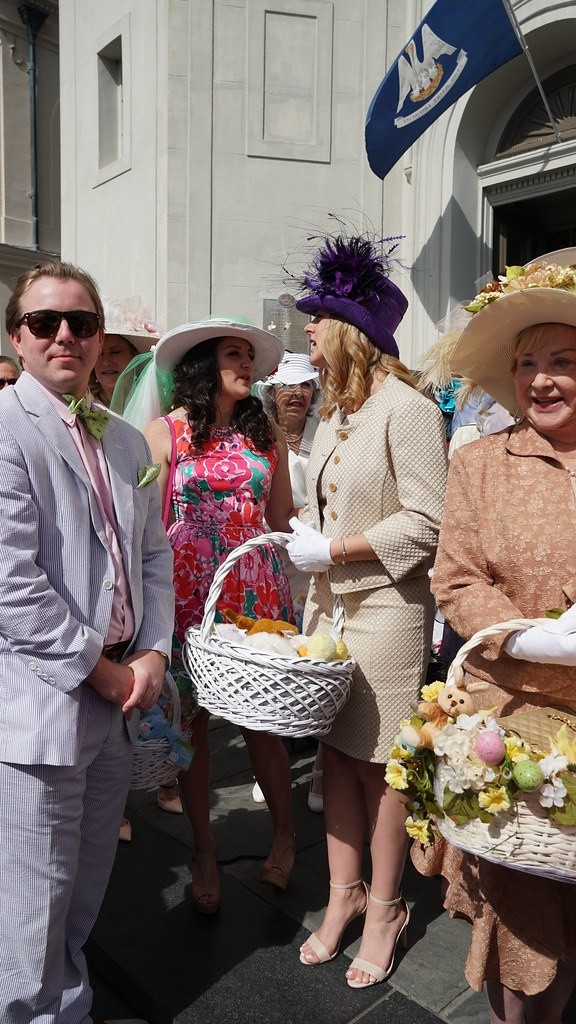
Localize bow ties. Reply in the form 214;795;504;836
62;391;110;441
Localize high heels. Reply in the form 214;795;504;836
258;832;296;891
299;877;372;965
347;890;410;989
191;834;221;915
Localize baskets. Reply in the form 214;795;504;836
181;532;356;738
433;618;576;884
128;670;193;792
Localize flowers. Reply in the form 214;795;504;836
384;683;576;847
460;261;576;313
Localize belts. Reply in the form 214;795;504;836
100;640;131;660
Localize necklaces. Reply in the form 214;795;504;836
207;424;240;433
565;466;576;478
285;431;304;443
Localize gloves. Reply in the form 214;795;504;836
286;516;338;572
503;603;576;666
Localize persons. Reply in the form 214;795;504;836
0;356;20;390
89;293;162;416
287;239;445;990
0;264;176;1024
144;317;293;918
259;353;326;634
408;246;576;1023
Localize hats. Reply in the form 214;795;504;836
88;294;161;396
521;247;576;268
296;230;409;360
251;352;327;416
154;318;285;384
449;262;576;418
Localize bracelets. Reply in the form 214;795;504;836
341;538;348;565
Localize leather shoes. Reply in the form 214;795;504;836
156;790;183;814
253;781;266;802
119;818;132;841
308;766;324;812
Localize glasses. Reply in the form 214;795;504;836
15;310;101;339
0;378;17;390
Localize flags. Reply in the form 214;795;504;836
364;1;526;179
